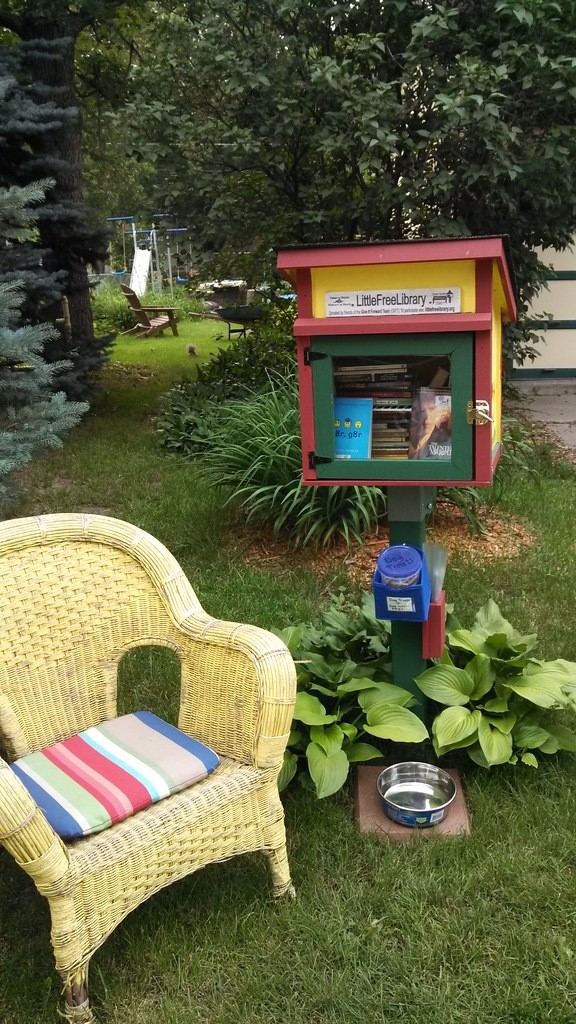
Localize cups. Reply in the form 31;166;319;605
376;544;422;589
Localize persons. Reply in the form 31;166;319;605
410;392;450;460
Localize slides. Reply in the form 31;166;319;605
128;246;153;299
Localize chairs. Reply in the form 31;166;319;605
0;514;298;1024
117;284;180;337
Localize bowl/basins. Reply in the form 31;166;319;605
376;762;457;828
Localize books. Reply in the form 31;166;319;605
332;364;451;460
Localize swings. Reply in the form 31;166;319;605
173;235;191;284
107;223;126;276
